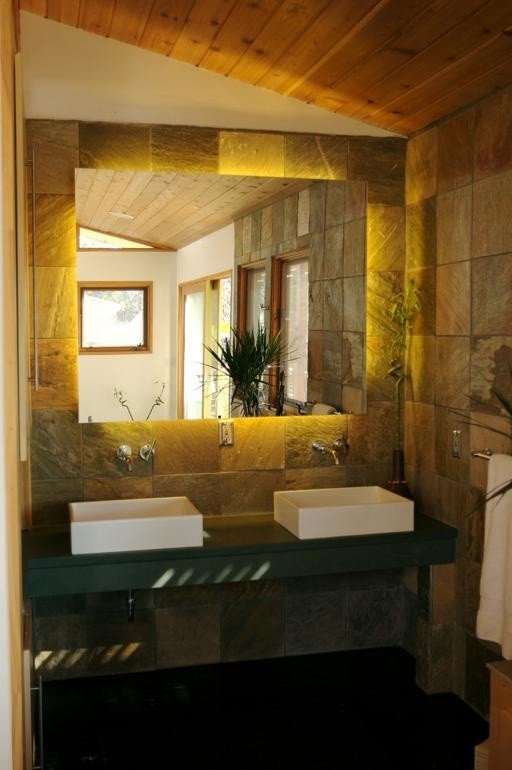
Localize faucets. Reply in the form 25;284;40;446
119;446;135;472
312;441;341;466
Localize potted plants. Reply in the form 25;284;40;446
382;272;423;497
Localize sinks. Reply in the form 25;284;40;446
66;494;203;554
274;484;417;541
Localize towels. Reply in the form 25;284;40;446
475;453;512;661
310;403;338;415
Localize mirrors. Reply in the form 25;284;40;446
78;167;370;424
